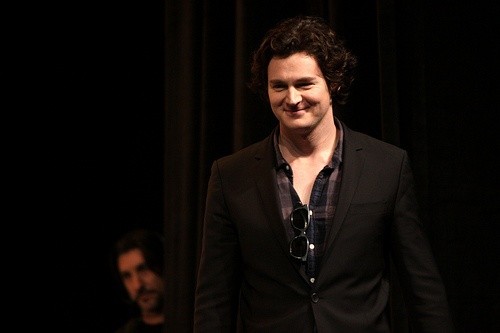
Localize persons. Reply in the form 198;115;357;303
193;15;454;333
106;228;168;333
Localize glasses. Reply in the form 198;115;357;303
290;203;311;261
